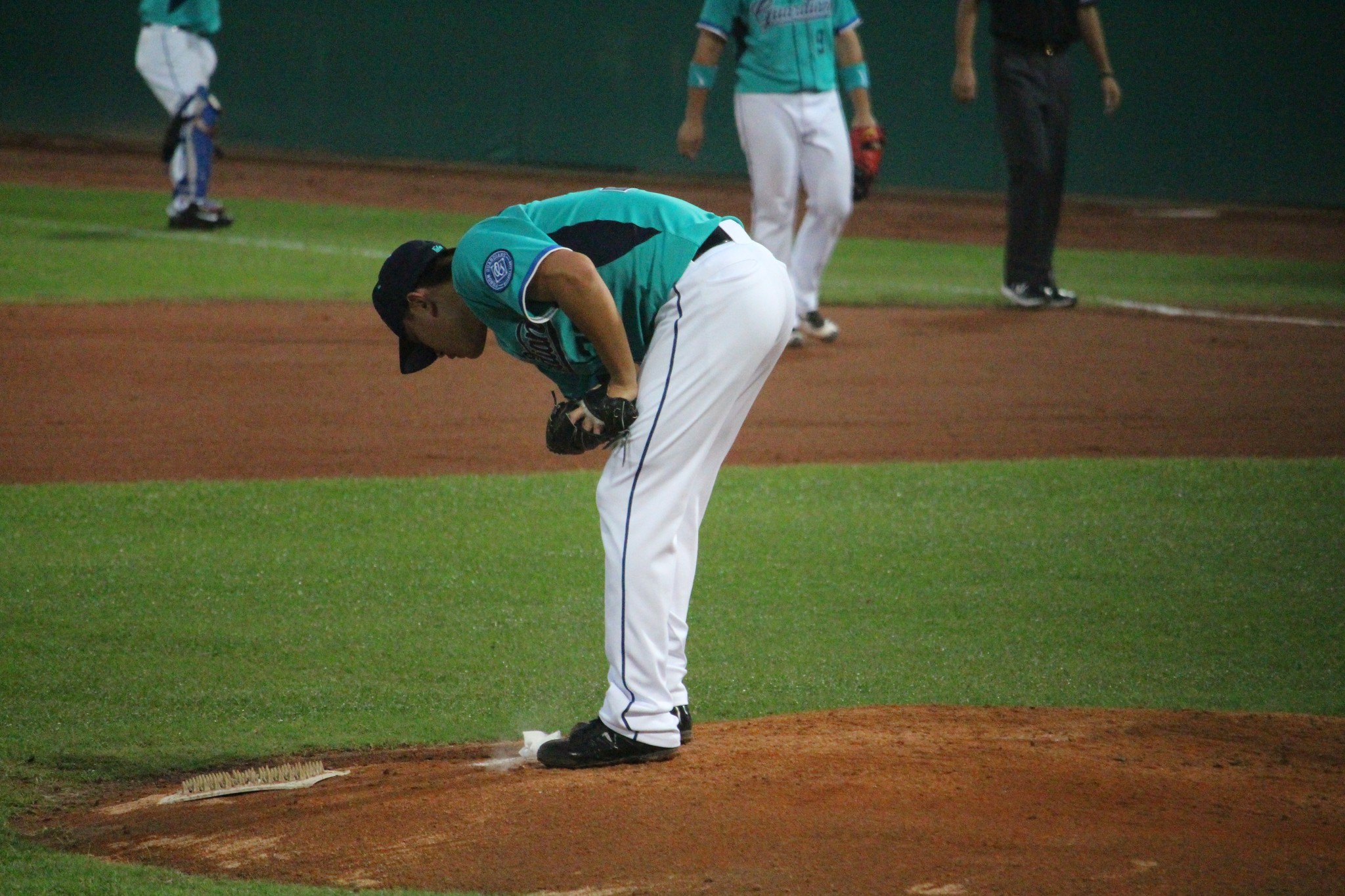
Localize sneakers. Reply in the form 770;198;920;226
1001;277;1047;306
570;704;692;741
791;329;806;345
536;716;679;768
801;310;840;340
1044;283;1077;307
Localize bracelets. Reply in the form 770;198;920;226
1097;70;1115;78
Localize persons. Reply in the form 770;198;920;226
370;184;797;768
953;0;1124;309
136;0;237;231
678;0;882;350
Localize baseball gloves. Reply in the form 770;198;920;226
546;389;639;455
849;125;885;202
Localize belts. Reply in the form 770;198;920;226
998;35;1064;56
690;226;733;262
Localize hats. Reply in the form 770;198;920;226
372;240;448;375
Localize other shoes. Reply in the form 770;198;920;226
170;203;234;229
